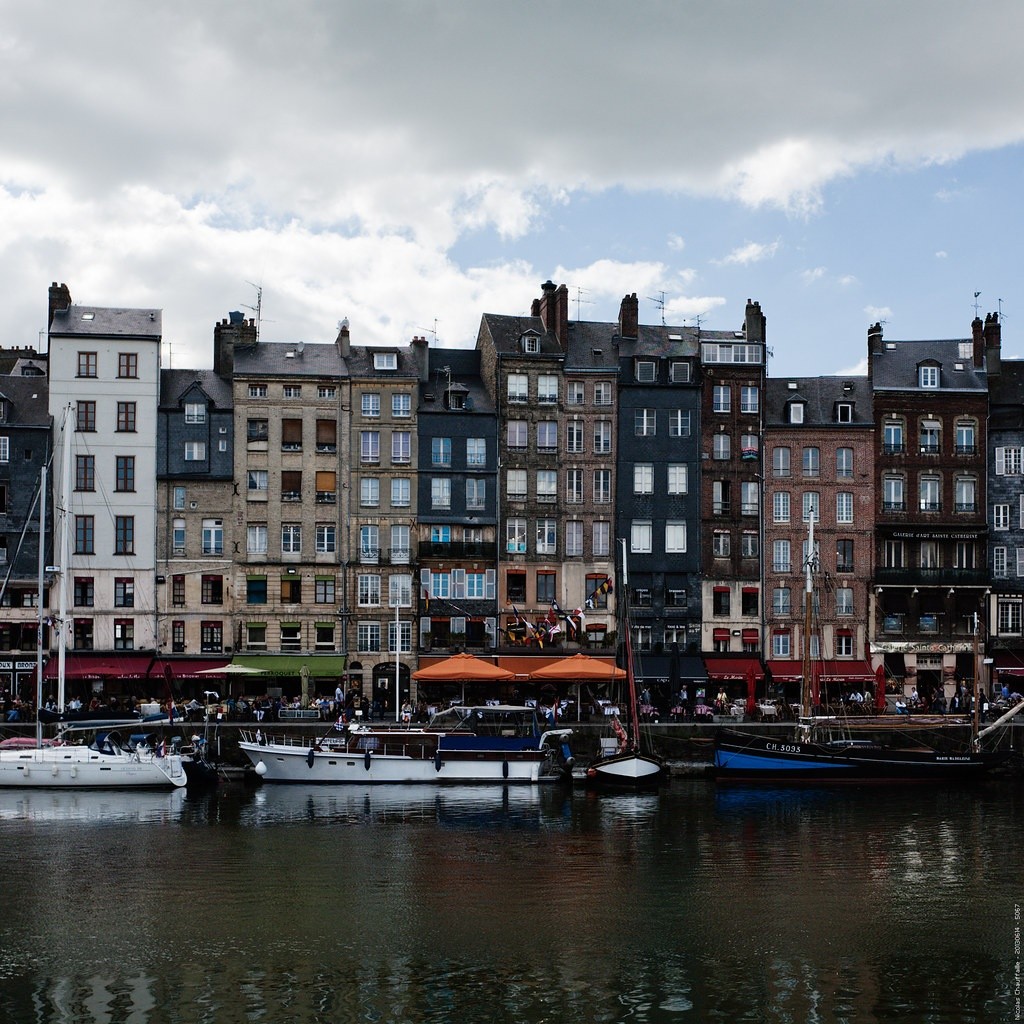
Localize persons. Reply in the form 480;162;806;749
400;697;446;723
64;695;302;723
190;733;206;754
637;684;746;724
820;688;873;715
897;677;1024;724
308;682;390;721
758;683;814;723
0;689;57;721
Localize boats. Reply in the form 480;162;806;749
237;705;618;783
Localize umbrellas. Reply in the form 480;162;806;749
300;664;311;708
810;667;820;714
747;663;757;719
413;653;515;717
529;654;626;722
164;663;173;707
875;664;886;713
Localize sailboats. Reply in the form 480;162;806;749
590;537;665;788
0;402;188;787
715;507;1000;784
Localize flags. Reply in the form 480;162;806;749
334;713;347;731
157;740;167;758
424;579;613;649
547;697;560;729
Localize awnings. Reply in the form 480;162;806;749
1002;670;1024;676
43;656;346;678
418;656;877;682
